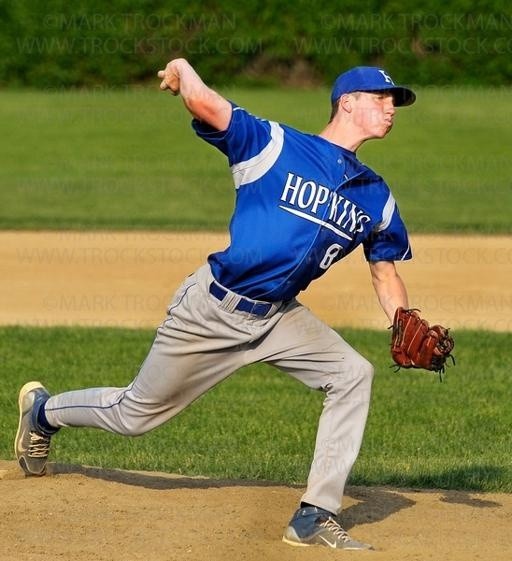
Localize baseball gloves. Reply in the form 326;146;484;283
390;308;454;372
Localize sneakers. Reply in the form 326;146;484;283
280;504;374;551
12;380;58;478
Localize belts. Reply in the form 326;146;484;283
207;278;290;318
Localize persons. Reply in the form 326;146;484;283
11;52;455;554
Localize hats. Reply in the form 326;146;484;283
331;66;418;110
